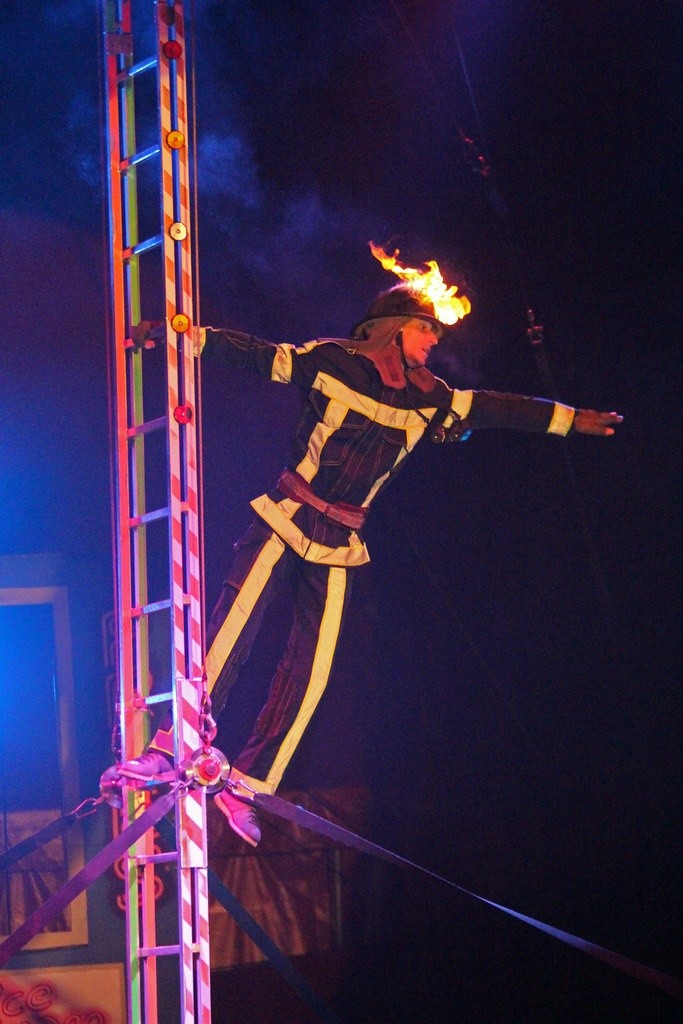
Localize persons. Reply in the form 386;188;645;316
119;284;624;845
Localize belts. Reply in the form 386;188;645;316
281;471;364;529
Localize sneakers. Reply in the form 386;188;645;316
117;748;174;780
214;788;261;847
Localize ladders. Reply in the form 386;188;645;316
106;0;212;1024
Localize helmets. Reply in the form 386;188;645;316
351;286;446;341
